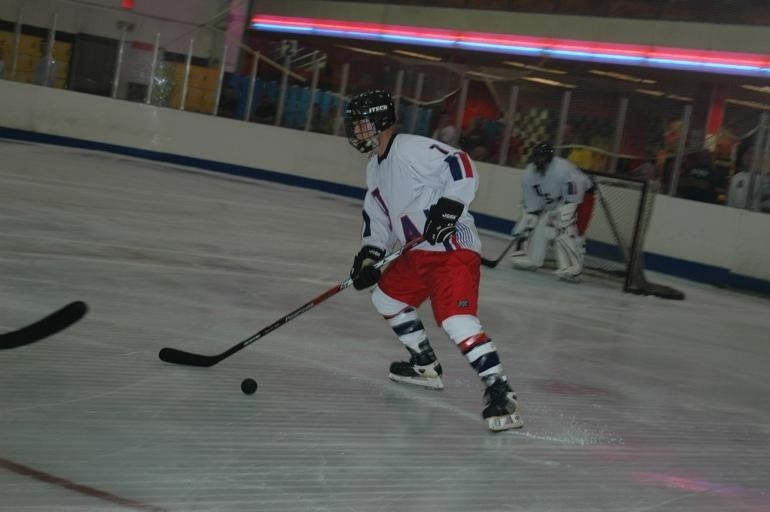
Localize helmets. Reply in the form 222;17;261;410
344;88;398;153
532;142;556;164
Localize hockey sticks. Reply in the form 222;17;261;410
480;228;528;268
158;235;429;367
0;300;87;349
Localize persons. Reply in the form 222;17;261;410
565;133;595;171
217;84;236;119
299;101;324;131
321;105;347;138
31;40;57;87
431;111;501;162
510;142;595;283
658;134;770;212
251;93;287;125
342;90;519;421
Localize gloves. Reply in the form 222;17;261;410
349;244;387;290
423;198;464;247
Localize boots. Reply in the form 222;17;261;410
481;373;520;420
389;342;443;379
